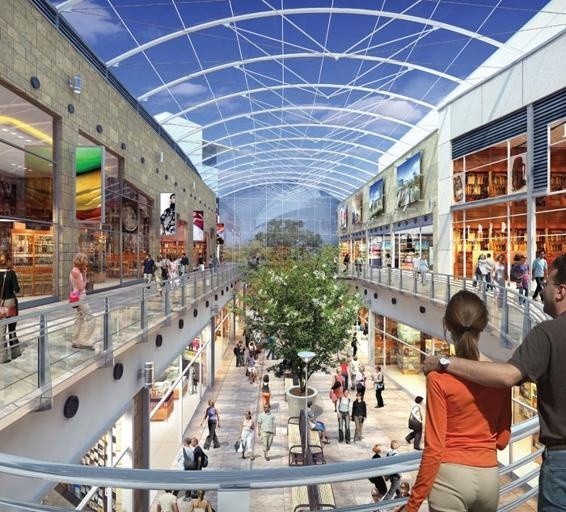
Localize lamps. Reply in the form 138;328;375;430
69;74;82;95
138;360;155;392
156;151;164;162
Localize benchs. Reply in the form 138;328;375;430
287;409;338;512
284;367;306;400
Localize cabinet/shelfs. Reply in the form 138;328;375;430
160;241;206;268
463;169;565;203
0;222;88;296
456;227;566;263
432;336;450;357
351;232;433;269
148;374;188;422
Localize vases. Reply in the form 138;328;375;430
285;387;318;418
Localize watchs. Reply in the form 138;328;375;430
438;354;451;373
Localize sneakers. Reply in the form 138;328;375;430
319;435;330;444
264;452;270;462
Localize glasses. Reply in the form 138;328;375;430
540;279;561;289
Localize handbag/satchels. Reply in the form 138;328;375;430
408;418;421;431
181;447;192;471
234;440;244;454
68;289;82;308
1;305;17;318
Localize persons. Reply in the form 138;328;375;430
141;251;221;296
160;194;175;235
401;290;512;512
343;250;549;302
0;252;22;364
421;252;566;512
155;320;276;512
305;326;424;512
68;251;96;351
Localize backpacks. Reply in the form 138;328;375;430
201;454;208;468
261;383;269;395
